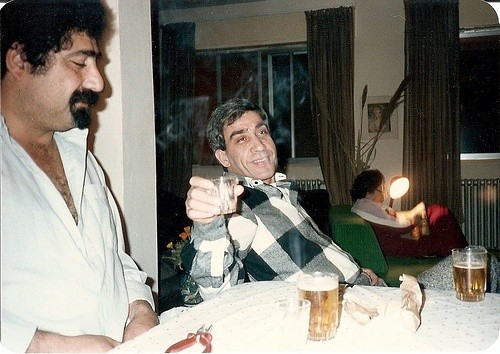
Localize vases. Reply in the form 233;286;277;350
178;268;204;307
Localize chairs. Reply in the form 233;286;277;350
419;244;500;293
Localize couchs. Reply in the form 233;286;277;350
329;204;441;287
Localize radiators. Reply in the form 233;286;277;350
287;178;324;190
460;178;499;250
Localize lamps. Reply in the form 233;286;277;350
388;175;410;208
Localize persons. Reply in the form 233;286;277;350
186;97;390;301
349;169;470;258
0;0;160;354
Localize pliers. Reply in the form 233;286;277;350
165;324;213;353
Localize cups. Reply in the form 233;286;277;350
272;298;310;351
296;271;341;341
210;177;237;214
452;246;487;302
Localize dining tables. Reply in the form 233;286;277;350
101;280;500;351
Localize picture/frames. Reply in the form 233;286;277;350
360;95;397;141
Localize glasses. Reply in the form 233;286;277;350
375;188;387;197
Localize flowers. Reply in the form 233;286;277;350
162;224;196;271
